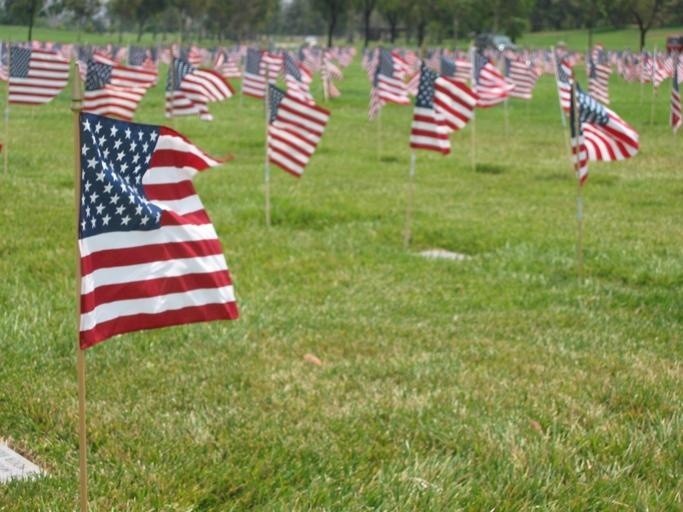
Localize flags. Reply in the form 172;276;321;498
78;110;238;349
